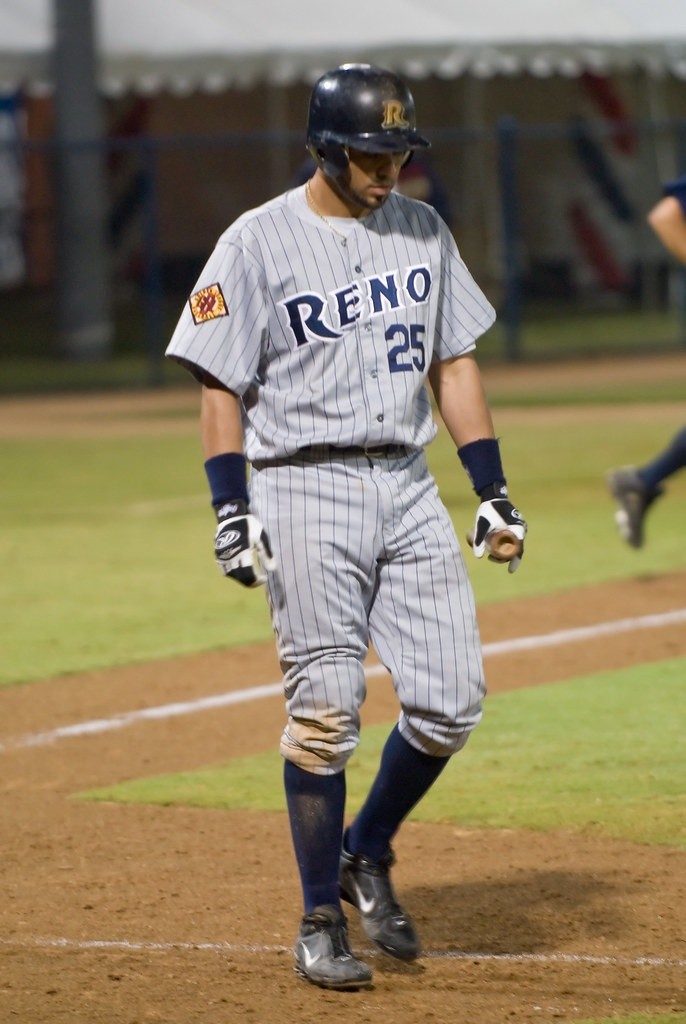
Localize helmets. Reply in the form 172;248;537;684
306;61;431;178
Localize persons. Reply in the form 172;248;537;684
164;63;529;989
391;140;686;548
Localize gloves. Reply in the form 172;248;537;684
472;481;528;573
214;497;277;588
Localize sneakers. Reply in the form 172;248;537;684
335;826;419;963
293;903;374;988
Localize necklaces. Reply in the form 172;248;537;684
305;177;370;246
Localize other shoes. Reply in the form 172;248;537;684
609;467;664;549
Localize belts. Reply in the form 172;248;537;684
300;442;406;456
